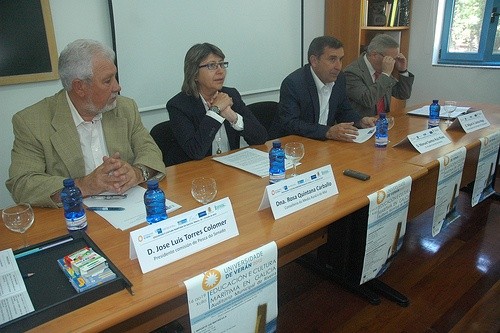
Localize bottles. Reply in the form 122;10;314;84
60;179;88;233
144;180;167;226
375;113;388;147
428;100;440;129
268;142;285;185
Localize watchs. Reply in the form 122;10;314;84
209;106;221;115
135;164;149;182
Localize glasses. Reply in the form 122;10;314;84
198;62;229;70
377;53;399;62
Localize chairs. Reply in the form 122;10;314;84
246;101;280;140
150;121;184;167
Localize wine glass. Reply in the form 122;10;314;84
1;203;35;249
191;177;217;205
284;142;304;178
385;116;395;143
443;100;456;124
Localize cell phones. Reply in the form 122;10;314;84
343;169;370;181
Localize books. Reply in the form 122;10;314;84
361;0;368;28
385;0;401;27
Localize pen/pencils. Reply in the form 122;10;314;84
86;207;124;211
22;273;35;278
90;194;128;199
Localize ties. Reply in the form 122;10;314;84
375;71;385;114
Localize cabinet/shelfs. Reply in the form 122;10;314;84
324;0;413;68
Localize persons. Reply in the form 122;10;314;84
5;39;167;209
166;42;268;163
344;34;414;117
268;36;375;142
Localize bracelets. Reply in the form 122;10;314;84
398;69;407;73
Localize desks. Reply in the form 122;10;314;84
0;134;429;333
325;100;500;308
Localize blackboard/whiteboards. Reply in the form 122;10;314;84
0;0;60;87
